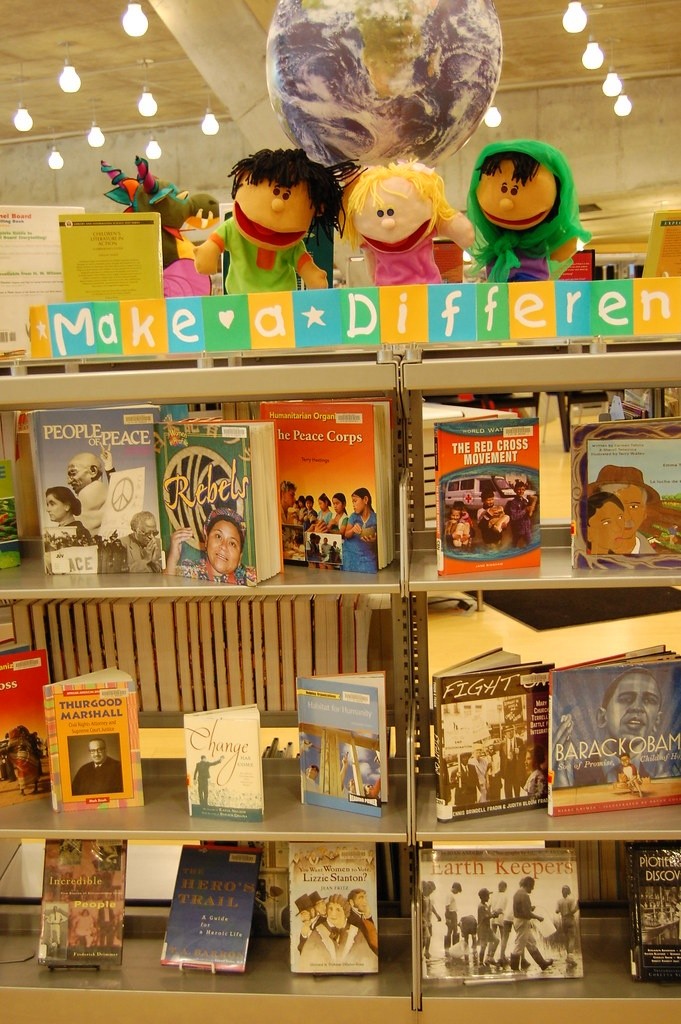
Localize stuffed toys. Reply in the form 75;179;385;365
100;156;219;298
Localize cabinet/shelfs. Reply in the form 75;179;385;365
0;341;680;1024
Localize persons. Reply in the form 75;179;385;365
280;481;378;574
193;139;592;293
72;909;93;948
0;643;387;974
98;899;114;945
364;750;380;798
300;739;319;792
340;751;356;797
450;726;549;805
444;481;533;550
422;876;580;970
163;507;257;587
43;441;162;574
47;906;68;949
72;739;124;796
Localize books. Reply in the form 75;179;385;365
219;202;333;295
623;839;681;984
596;210;681;278
160;402;260;420
558;249;596;281
417;847;583;985
260;398;395;574
432;647;555;823
59;212;163;302
549;644;681;815
156;418;284;587
571;387;681;570
433;417;541;576
432;238;463;283
0;458;21;569
29;403;162;574
